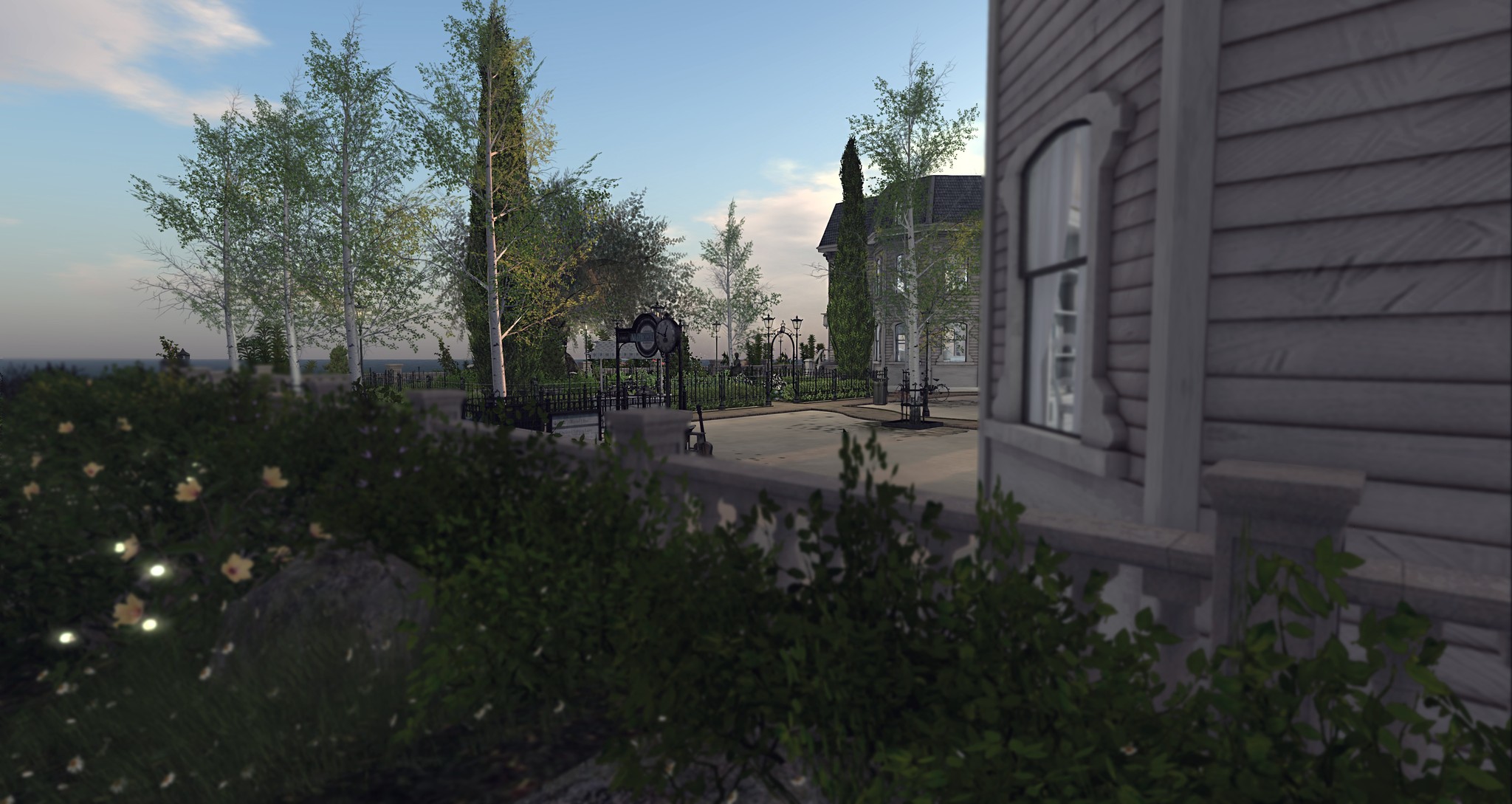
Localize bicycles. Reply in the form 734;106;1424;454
896;378;950;402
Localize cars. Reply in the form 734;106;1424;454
619;359;629;366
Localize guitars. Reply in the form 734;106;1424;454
693;405;713;455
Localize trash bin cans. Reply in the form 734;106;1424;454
871;378;889;405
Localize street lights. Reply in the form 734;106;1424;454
648;299;667;394
354;300;367;390
919;307;935;417
776;330;784;363
761;313;776;407
790;315;803;404
580;318;591;379
610;316;624;410
711;320;722;375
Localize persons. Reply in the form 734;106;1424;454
732;353;742;377
